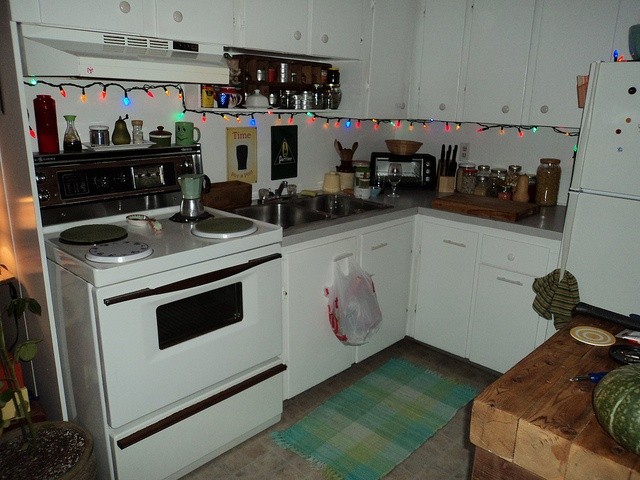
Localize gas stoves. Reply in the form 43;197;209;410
44;203;282;277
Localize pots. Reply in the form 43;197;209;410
149;125;171;148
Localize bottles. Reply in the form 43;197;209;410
131;121;143;143
291;73;297;83
33;94;60;155
326;83;342;110
63;115;82;151
332;75;335;83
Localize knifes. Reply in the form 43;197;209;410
440;143;458;174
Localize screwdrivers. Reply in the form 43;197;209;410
573;372;607;383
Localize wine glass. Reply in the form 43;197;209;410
387;163;402;198
373;161;384;193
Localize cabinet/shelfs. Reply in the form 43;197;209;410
0;0;236;47
467;227;553;375
281;215;416;403
237;0;366;61
406;214;482;359
460;0;621;129
411;0;469;122
317;0;418;120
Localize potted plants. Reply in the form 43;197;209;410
0;263;98;480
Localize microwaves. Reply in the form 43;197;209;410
371;153;437;194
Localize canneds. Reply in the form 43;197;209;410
201;86;214;107
329;67;340;84
457;164;477;195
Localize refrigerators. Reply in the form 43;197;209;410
535;60;640;349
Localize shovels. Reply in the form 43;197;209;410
331;139;360;163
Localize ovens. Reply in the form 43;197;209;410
96;238;282;431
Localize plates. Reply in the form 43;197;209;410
82;141;155;151
243;104;272;110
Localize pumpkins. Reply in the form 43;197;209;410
593;362;640;455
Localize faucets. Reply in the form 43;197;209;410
275;181;287;197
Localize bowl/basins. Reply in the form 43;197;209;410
291;91;314;110
385;140;423;155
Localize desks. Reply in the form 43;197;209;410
471;311;640;479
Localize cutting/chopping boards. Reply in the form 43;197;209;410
433;193;539;223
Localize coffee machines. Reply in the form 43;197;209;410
178;174;211;220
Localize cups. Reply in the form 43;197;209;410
175;122;201;146
362;189;371;200
220;87;241;108
125;215;151;252
353;188;363;200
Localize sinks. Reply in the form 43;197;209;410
302;196;382;214
237;203;328;229
